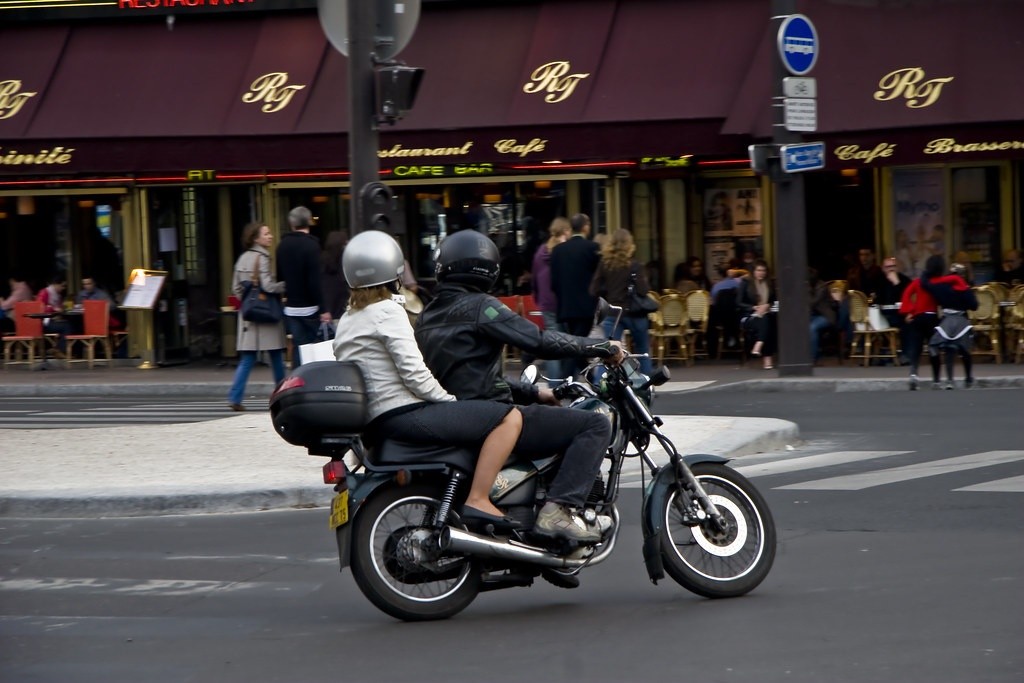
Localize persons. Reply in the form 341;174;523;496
36;277;76;358
897;255;979;392
806;267;837;365
318;231;350;333
331;230;523;531
526;210;653;389
0;271;33;333
737;261;779;369
672;255;754;352
988;248;1024;289
412;229;626;589
224;223;288;412
277;205;331;368
848;246;885;299
73;278;116;306
863;257;913;365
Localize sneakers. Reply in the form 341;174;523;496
533;505;601;543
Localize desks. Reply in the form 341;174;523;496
23;313;58;371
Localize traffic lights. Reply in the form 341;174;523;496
376;64;427;118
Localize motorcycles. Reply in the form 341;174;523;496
266;294;777;622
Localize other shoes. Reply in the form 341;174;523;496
228;400;247;411
931;382;943;391
763;365;774;370
750;350;761;355
945;380;955;390
908;375;919;391
459;504;522;529
965;377;976;388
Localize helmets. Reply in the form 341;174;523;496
342;230;405;289
432;228;501;293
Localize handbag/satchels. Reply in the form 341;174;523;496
616;262;660;318
298;321;335;365
240;253;288;324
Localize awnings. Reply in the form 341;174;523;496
1;0;1024;183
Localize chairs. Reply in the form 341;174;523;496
643;289;710;369
496;295;545;361
829;280;900;367
966;281;1024;365
2;301;45;370
64;300;112;369
